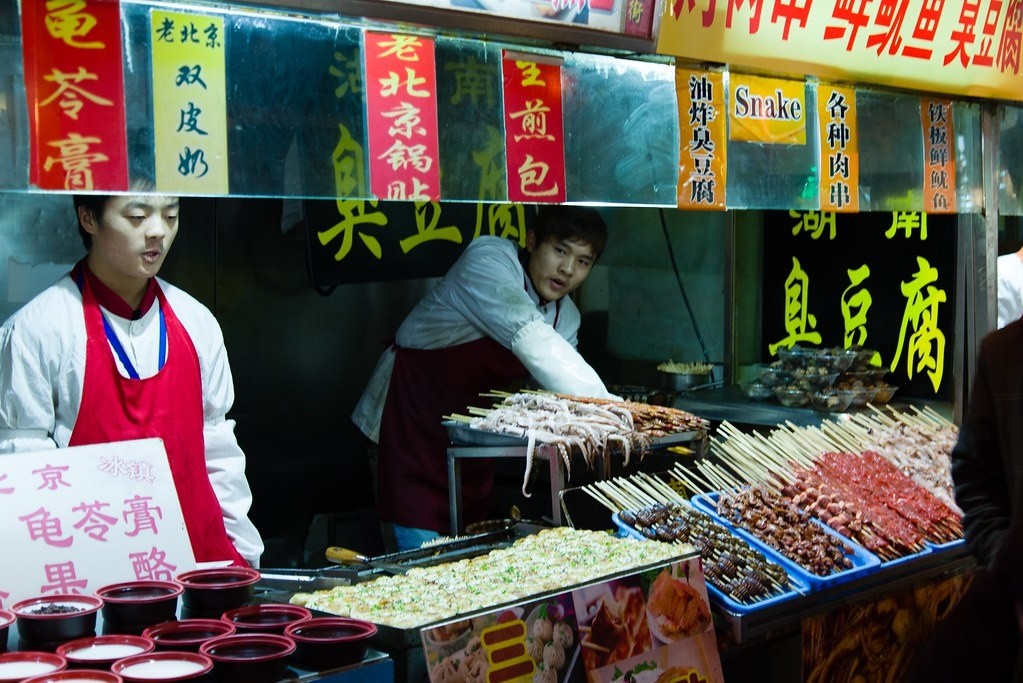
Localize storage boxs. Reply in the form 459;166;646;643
612;501;811;615
691;489;881;590
880;536;969;570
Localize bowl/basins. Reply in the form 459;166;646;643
426;625;471;657
0;567;378;683
737;346;899;413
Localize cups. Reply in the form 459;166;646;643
657;370;709;394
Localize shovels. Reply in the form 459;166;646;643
326;546;416;575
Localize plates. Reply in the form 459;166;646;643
646;607;713;644
494;606;524;620
524;603;563;637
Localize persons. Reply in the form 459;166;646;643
997;245;1023;328
351;205;623;548
0;128;265;570
952;319;1023;682
